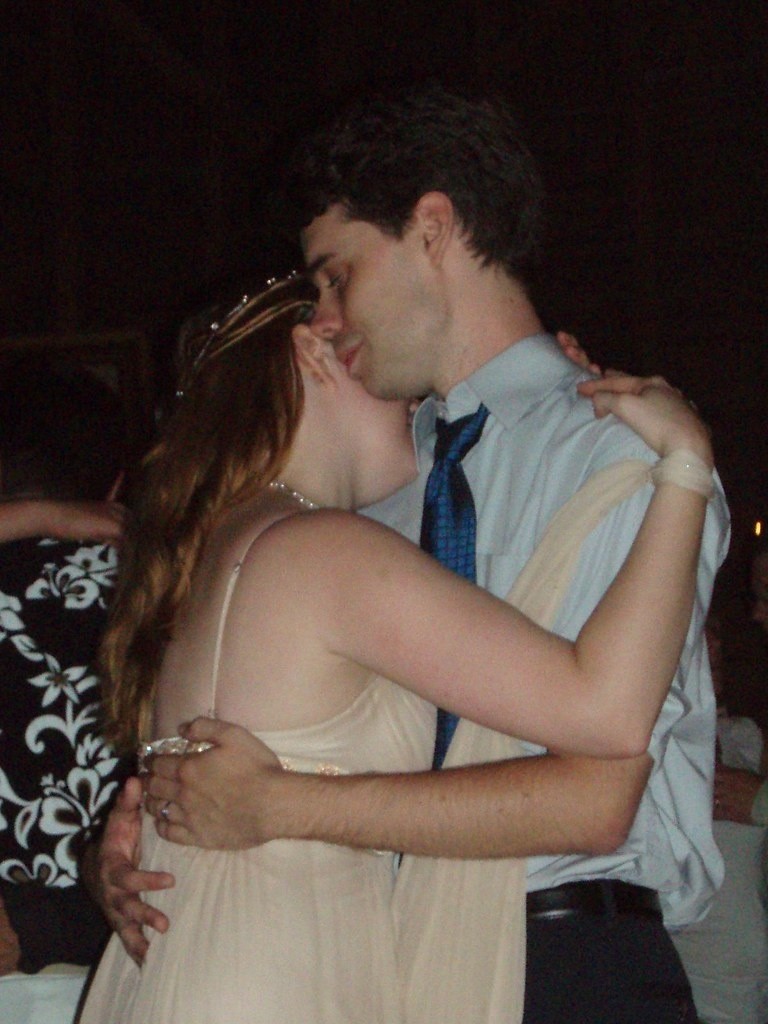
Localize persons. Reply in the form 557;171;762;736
78;266;717;1024
672;526;768;1024
0;372;138;972
83;70;730;1024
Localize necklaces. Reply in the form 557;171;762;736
266;479;319;511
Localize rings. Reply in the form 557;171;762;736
162;801;171;819
715;799;720;807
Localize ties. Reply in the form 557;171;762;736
419;401;490;770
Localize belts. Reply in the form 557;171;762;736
525;879;663;926
